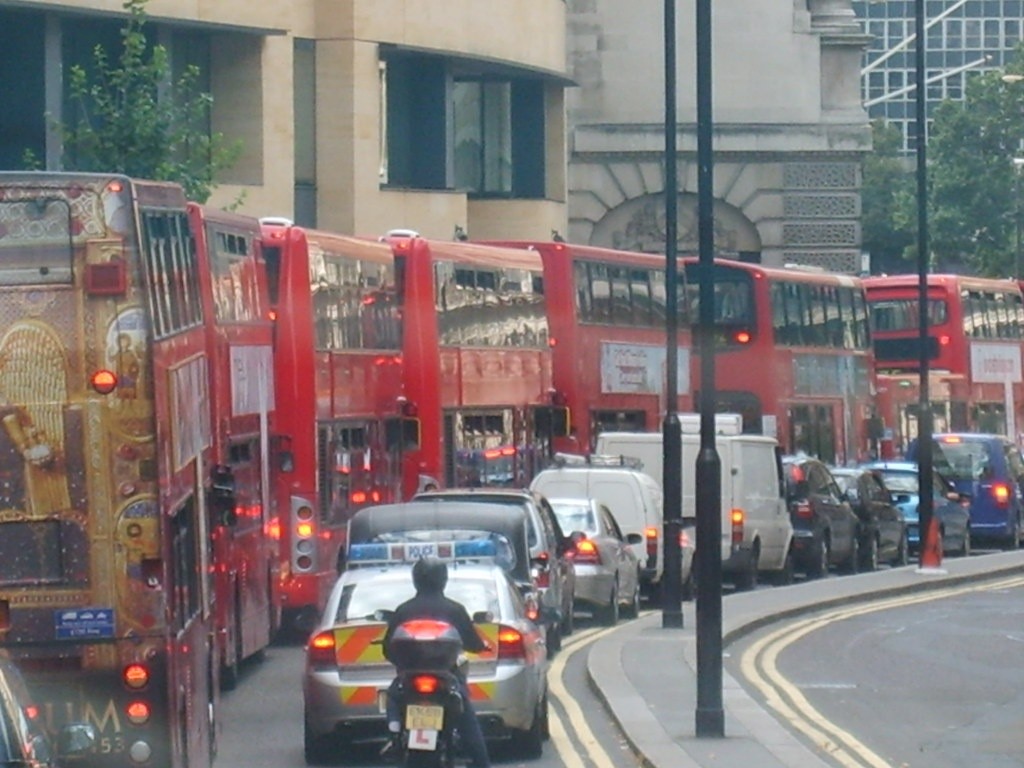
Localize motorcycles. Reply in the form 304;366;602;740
371;609;495;768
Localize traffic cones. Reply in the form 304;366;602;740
912;516;946;577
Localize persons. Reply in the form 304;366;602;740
383;558;492;768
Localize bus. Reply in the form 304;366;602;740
843;271;1023;452
187;201;274;695
259;216;417;638
462;239;694;457
682;257;884;473
0;171;223;767
380;228;574;496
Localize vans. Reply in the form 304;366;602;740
594;410;812;595
902;432;1024;552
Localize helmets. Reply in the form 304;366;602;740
412;557;447;590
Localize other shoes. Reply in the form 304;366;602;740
380;739;401;757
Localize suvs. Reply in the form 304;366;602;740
527;452;701;610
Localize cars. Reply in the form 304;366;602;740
822;466;912;571
304;530;561;763
0;647;95;768
333;502;575;658
865;459;975;561
539;495;643;626
408;486;589;639
776;453;868;582
